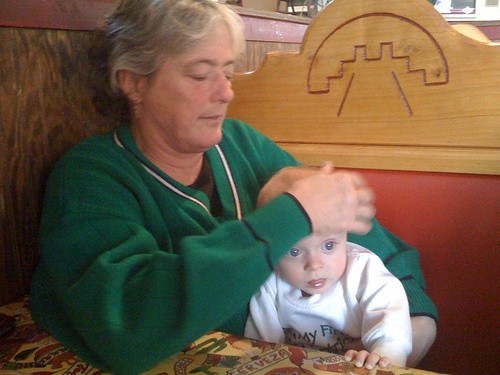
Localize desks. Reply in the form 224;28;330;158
0;296;442;375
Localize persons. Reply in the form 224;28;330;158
23;1;443;375
242;166;414;371
